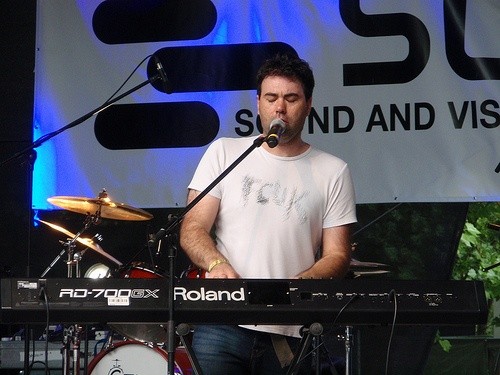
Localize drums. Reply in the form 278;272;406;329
109;262;172;347
86;341;195;375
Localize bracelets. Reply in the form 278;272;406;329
207;258;230;273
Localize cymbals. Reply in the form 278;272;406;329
47;196;153;221
37;219;122;267
349;258;389;276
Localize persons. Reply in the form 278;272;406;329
179;54;357;375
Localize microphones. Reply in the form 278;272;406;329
266;118;286;148
154;58;173;94
148;225;159;258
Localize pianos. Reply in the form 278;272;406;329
0;277;487;325
